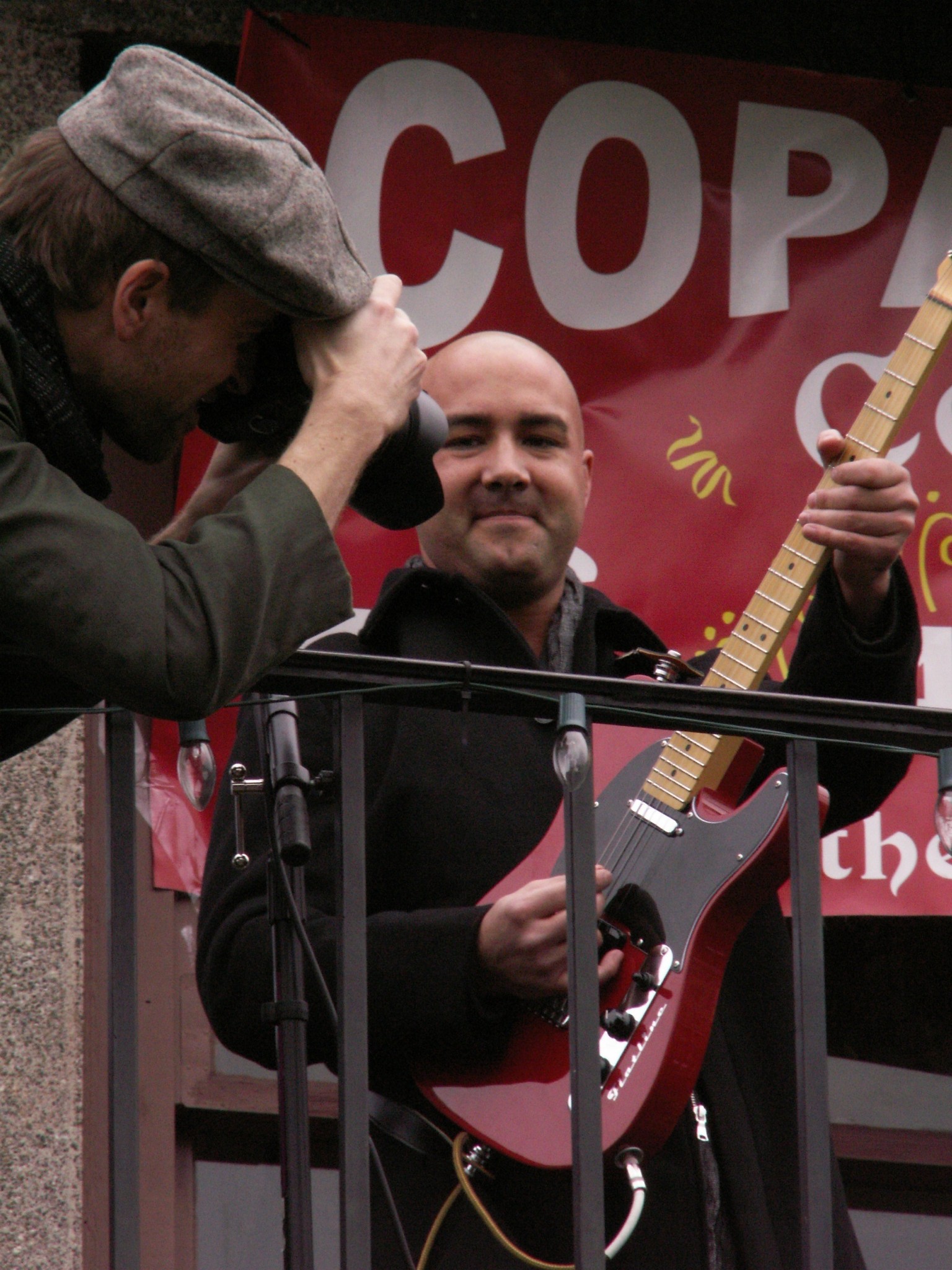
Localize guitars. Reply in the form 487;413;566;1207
412;252;952;1170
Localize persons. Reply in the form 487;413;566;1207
194;330;925;1270
0;44;430;776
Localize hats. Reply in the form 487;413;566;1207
58;42;366;320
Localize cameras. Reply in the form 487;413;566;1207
195;312;452;531
